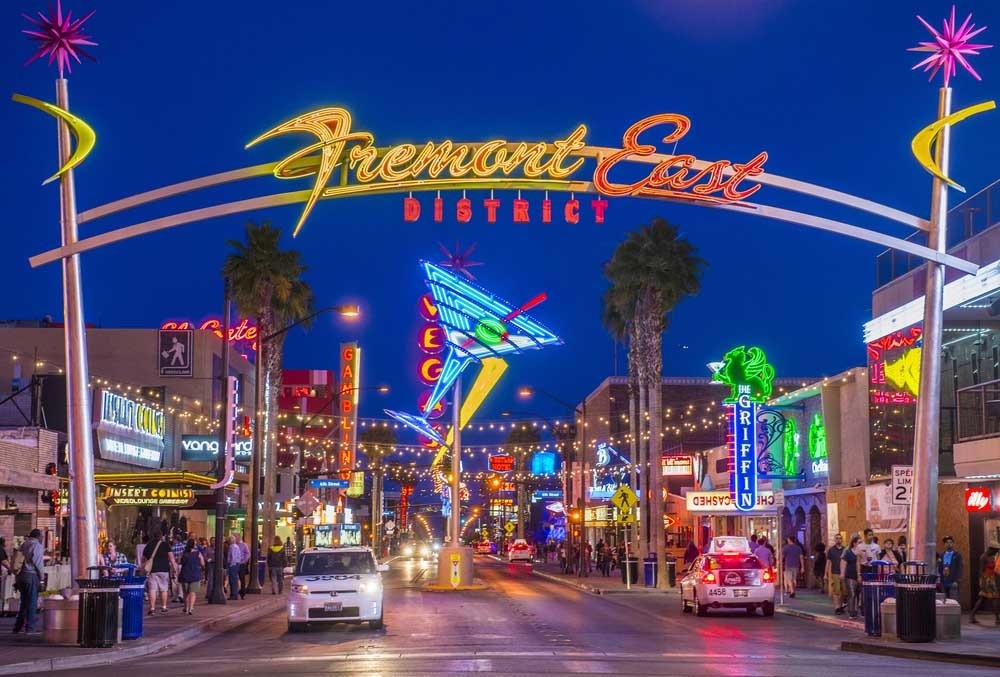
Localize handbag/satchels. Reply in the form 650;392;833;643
140;558;153;576
9;551;26;575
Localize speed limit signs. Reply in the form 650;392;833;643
891;464;913;505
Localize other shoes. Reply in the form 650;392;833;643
241;595;247;600
189;611;193;616
860;612;865;618
842;602;851;607
160;607;171;614
836;608;841;615
148;610;156;616
25;629;44;635
12;629;25;634
849;614;858;620
182;609;189;614
226;595;238;600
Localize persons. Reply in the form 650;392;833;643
0;525;296;635
683;529;1000;626
532;539;628;579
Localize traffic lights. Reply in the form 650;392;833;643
571;509;580;523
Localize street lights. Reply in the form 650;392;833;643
503;411;573;574
245;305;358;593
294;386;388;565
474;508;506;556
520;387;588;577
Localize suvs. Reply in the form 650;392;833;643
283;544;390;632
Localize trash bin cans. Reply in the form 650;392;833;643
102;563;148;640
665;552;676;587
74;566;123;648
643;552;658;588
891;559;939;642
620;552;639;584
258;556;266;587
858;559;894;637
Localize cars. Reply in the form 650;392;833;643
509;543;532;562
402;537;441;560
680;551;775;617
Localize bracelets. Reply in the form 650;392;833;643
40;581;44;584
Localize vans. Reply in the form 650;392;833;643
479;542;491;553
